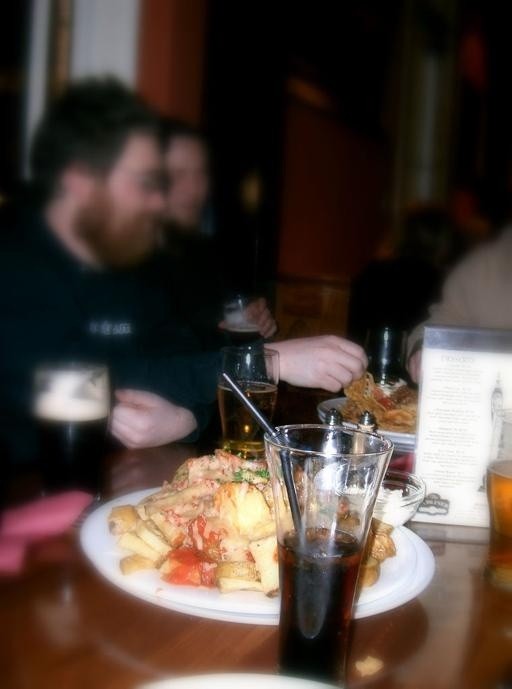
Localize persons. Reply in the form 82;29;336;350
347;85;512;410
2;71;378;523
105;120;282;357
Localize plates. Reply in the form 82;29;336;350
80;482;435;626
316;382;417;454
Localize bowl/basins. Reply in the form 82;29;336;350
368;472;427;528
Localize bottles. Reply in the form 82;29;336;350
26;268;113;500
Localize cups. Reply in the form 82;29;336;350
224;301;264;360
265;424;394;683
366;326;409;384
218;348;278;463
486;408;512;545
